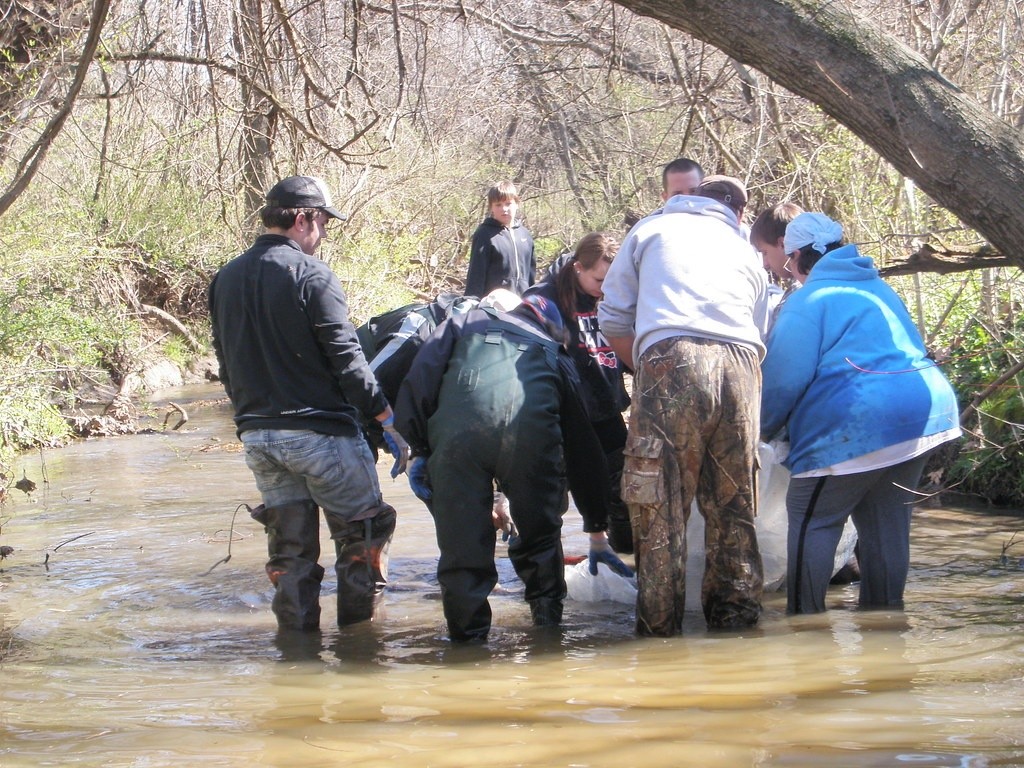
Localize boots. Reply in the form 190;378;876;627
322;500;396;626
248;497;325;631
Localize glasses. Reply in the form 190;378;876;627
783;257;792;272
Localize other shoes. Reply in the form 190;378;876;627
830;562;860;584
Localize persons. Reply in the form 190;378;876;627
329;290;521;581
393;288;631;645
634;155;753;243
525;233;646;556
594;175;770;634
465;183;535;304
208;173;408;631
748;200;860;583
760;213;963;617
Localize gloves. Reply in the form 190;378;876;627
588;536;634;578
494;493;519;545
409;457;433;499
382;424;409;479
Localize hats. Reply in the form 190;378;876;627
266;175;347;221
696;174;747;207
